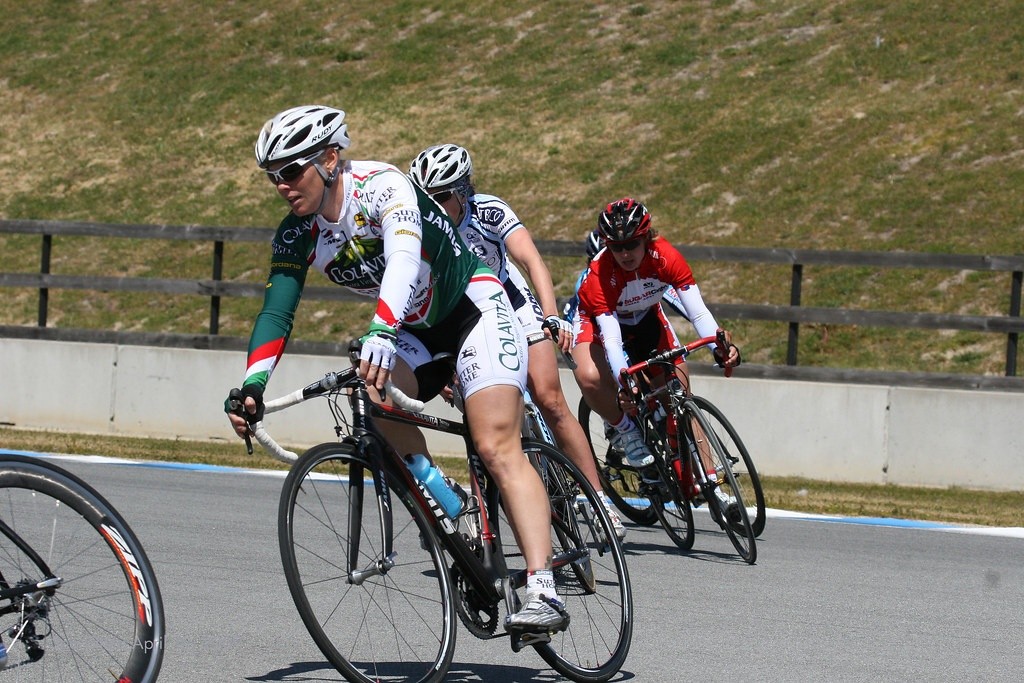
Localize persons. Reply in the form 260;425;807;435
224;104;627;632
563;200;743;524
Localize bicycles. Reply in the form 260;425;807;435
227;328;767;683
577;327;767;567
1;421;166;683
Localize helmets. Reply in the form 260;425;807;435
409;144;471;192
597;198;651;242
255;104;351;169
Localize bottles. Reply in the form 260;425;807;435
403;454;462;518
667;412;677;451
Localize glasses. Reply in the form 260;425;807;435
608;236;648;253
430;185;466;203
265;145;340;185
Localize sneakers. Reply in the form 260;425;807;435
503;591;570;635
621;431;655;468
590;506;626;542
605;422;626;454
717;493;742;522
